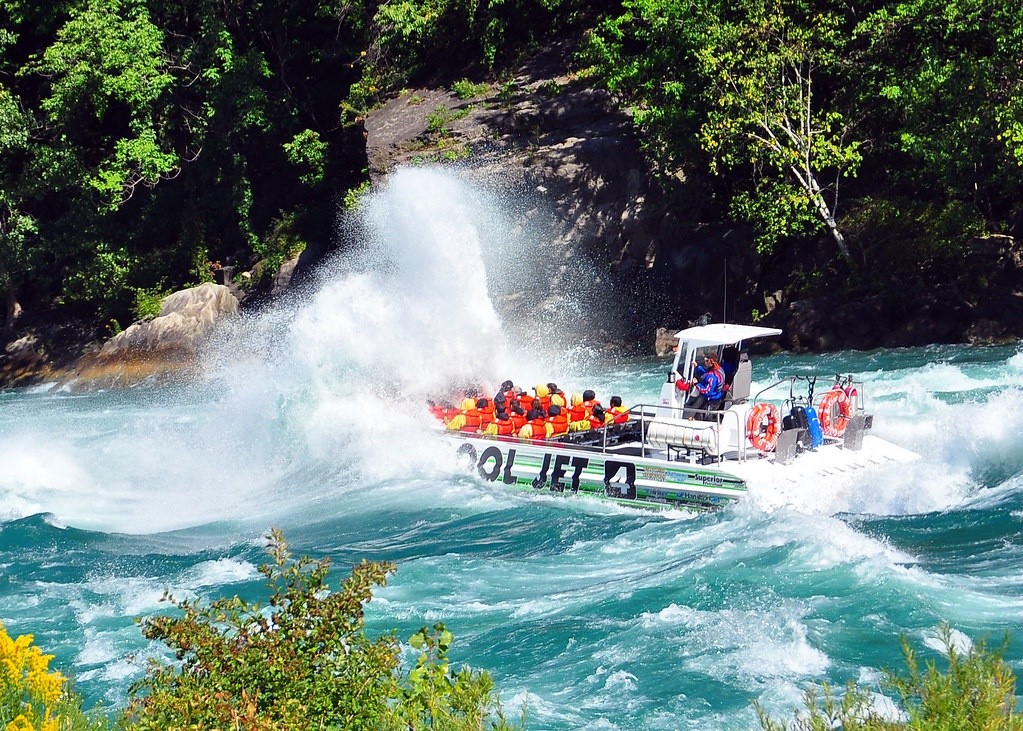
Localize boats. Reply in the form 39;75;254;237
430;322;923;506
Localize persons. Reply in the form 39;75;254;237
691;351;727;419
426;378;631;439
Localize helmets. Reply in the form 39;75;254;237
460;397;475;411
571;392;583;406
536;384;549;397
593;404;602;415
551;394;564;406
475;399;488;408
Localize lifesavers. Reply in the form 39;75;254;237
817;388;855;438
746;401;784;453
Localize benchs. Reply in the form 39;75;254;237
548;420;642;444
